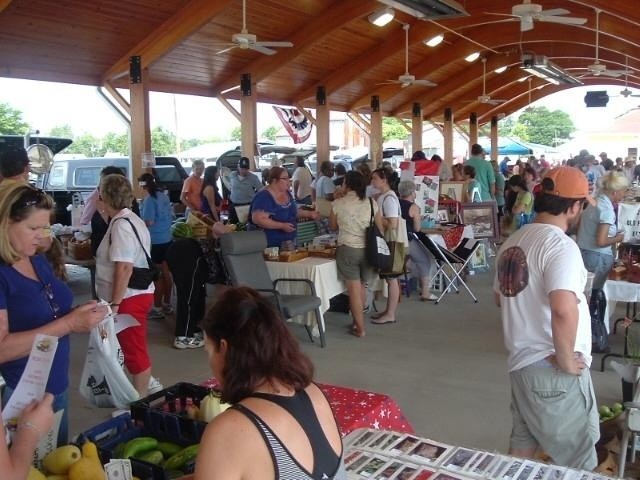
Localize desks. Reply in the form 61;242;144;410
600;253;640;372
134;373;415;438
263;256;389;343
62;253;98;301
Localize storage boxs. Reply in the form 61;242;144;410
185;210;216;225
191;226;213;237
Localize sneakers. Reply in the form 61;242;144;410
174;336;207;349
149;374;164;394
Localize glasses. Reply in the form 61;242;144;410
277;177;289;181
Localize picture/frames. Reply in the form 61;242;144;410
468;237;490;275
460;200;500;243
437;208;450;225
439;181;466;204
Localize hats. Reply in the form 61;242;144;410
540;166;597;207
238;157;250;169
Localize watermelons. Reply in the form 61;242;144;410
170;222;193;240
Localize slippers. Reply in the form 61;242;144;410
371;310;397;325
420;292;440;301
349;326;368;337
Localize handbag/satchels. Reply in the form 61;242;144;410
315;196;337;220
362;223;396;271
79;298;141;408
518;210;537;231
113;266;158;290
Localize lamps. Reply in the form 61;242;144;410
377;0;472;21
367;7;395;28
519;54;585;86
422;33;444;48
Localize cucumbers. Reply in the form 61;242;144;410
110;438;200;470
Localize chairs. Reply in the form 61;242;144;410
408;230;481;304
617;381;640;479
214;230;326;350
398;254;413;298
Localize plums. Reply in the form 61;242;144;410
597;402;624;421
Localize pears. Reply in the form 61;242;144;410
27;435;107;480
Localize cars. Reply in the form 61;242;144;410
420;142;445;161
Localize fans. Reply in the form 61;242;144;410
375;24;439;89
563;9;634;80
460;58;507;106
482;0;588;31
215;0;294;55
608;56;640;98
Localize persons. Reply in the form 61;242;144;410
1;139;639;480
